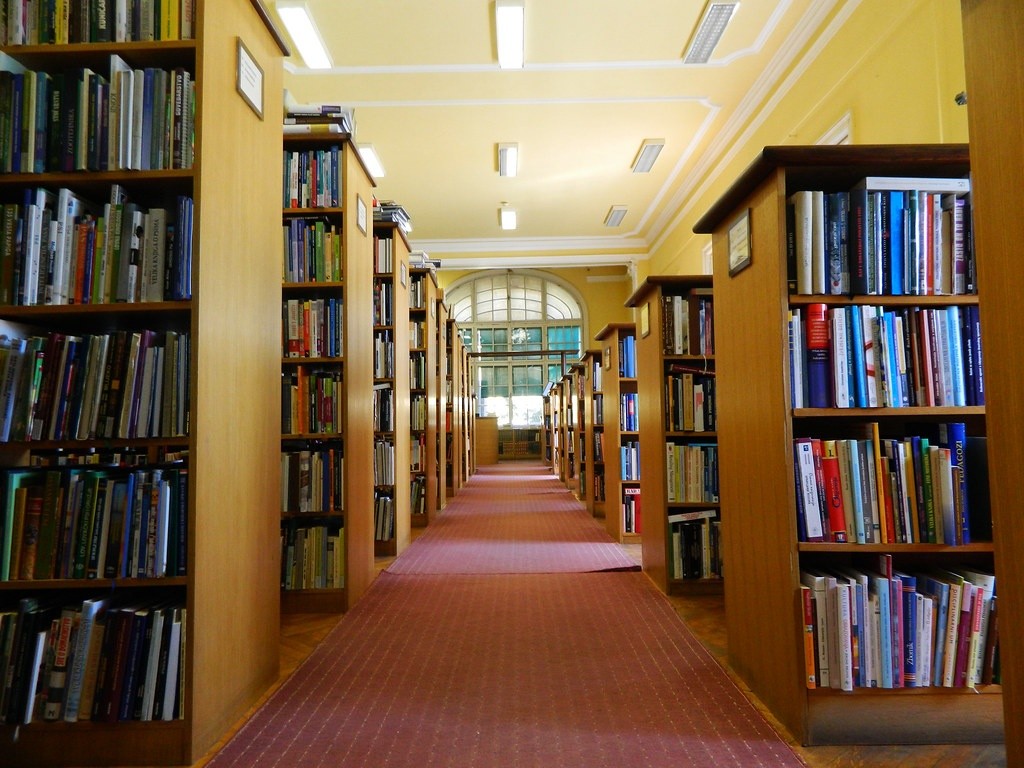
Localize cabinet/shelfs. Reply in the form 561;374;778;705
372;218;476;557
541;361;586;502
624;274;723;596
282;134;379;613
961;0;1024;767
579;349;606;518
691;141;1004;749
593;323;641;544
499;429;541;460
2;1;291;766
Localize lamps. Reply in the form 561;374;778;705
682;0;739;65
604;205;628;228
632;139;665;174
356;143;386;178
498;142;518;177
274;0;334;71
500;207;517;230
494;0;525;71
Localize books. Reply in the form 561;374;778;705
766;170;1004;706
0;0;199;767
373;199;412;542
651;287;718;585
408;251;454;515
544;327;641;529
283;105;351;589
